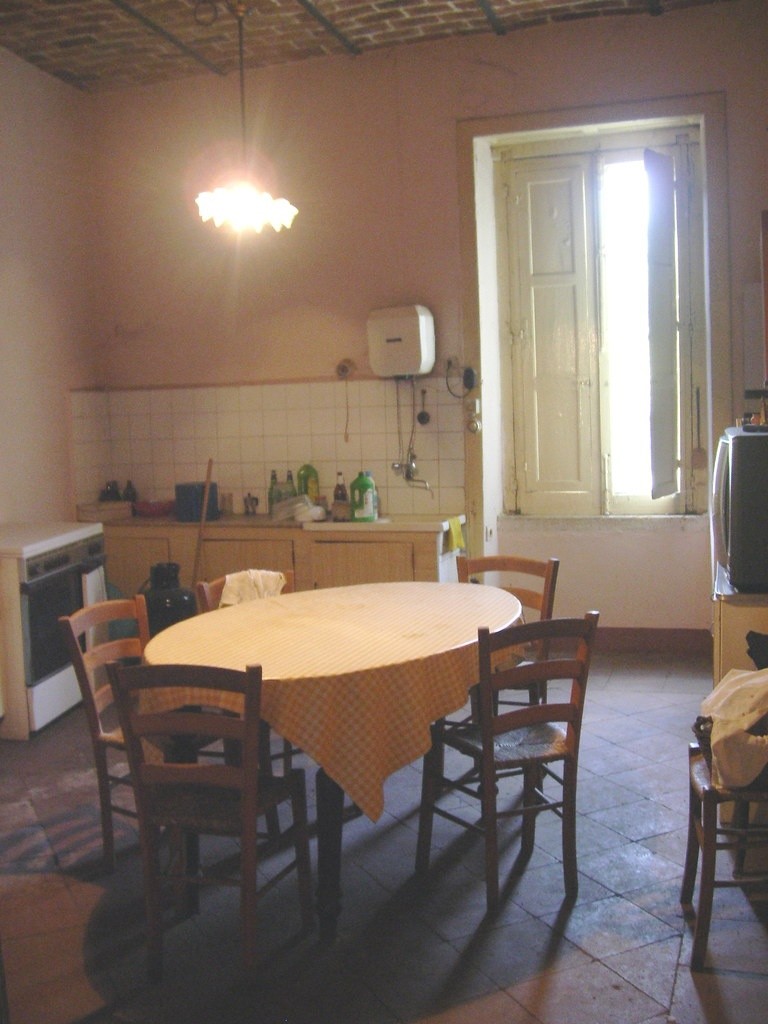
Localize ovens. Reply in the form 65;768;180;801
0;519;110;741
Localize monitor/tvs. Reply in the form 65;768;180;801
714;427;768;594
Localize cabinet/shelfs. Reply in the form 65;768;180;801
304;530;462;591
712;594;768;692
104;526;191;599
191;527;303;613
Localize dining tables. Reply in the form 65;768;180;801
144;581;523;946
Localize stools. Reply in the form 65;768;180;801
680;743;768;946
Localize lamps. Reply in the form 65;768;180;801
196;0;299;234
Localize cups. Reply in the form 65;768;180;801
221;493;233;515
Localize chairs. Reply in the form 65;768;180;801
103;660;316;954
434;555;560;799
417;610;611;909
194;569;305;776
57;594;226;876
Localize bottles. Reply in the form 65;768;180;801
350;471;373;522
281;471;296;500
334;472;346;521
123;481;137;516
268;470;281;514
365;471;378;521
297;461;319;505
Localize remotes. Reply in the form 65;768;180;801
743;424;768;432
